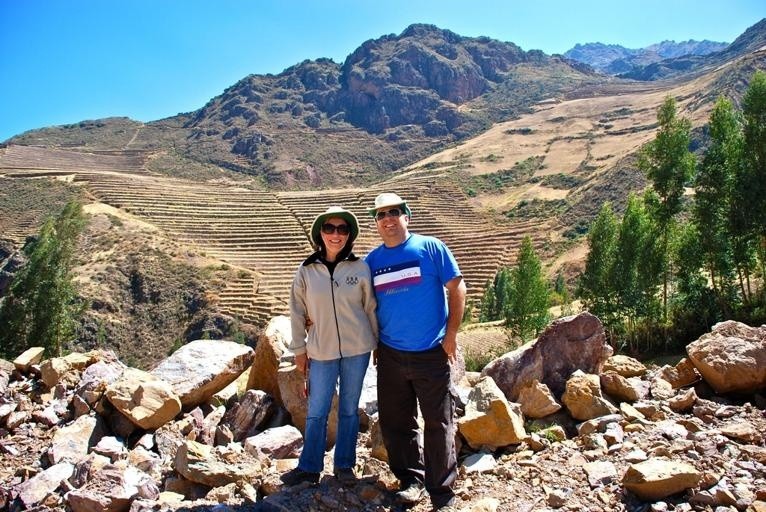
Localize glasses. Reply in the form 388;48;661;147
376;209;403;219
321;223;350;235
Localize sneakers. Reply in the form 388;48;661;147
334;467;356;487
279;468;320;488
431;485;458;510
396;484;425;504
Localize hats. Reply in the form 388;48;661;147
311;206;359;246
366;193;412;217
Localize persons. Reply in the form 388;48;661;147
279;207;382;487
361;193;467;511
